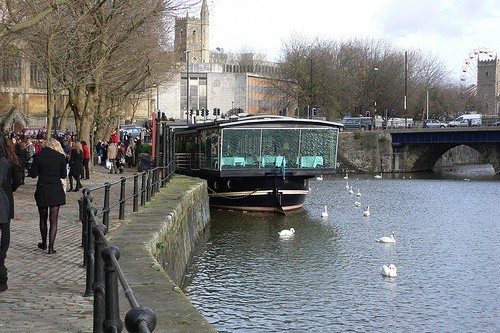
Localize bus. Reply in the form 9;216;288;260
386;117;413;129
337;114;373;133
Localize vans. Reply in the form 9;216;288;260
448;114;483;128
19;127;45;153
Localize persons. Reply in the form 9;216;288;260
423;120;429;128
0;130;23;293
382;120;387;129
6;128;90;192
371;114;375;131
28;138;67;254
282;138;289;150
90;125;141;174
144;122;152;143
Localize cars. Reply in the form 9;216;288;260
486;119;500;127
420;118;448;129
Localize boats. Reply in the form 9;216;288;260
165;114;344;217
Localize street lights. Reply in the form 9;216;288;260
423;91;429;120
186;49;209;125
53;84;58;134
156;75;163;110
303;55;313;109
374;67;379;116
148;86;155;117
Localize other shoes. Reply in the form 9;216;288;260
49;249;56;254
38;243;47;250
73;188;79;191
67;188;73;191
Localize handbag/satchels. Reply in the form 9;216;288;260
105;159;111;168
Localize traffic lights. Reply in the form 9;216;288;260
217;109;220;115
206;110;209;115
201;109;204;116
213;108;216;115
238;108;240;113
189;108;193;116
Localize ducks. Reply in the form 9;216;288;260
363;206;371;216
278;228;296;236
321;205;328;217
381;264;397;277
375;231;396;243
316;172;383;207
